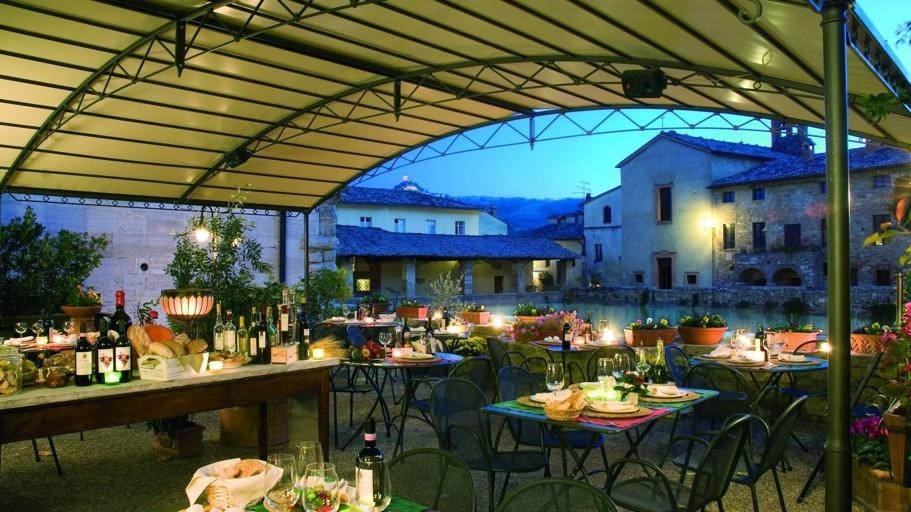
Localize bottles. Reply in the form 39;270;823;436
0;337;76;397
354;301;361;321
426;317;433;338
562;314;572;349
355;417;385;508
76;290;133;386
634;335;669;384
213;288;310;365
754;323;770;362
402;316;411;347
585;311;592;334
441;307;449;331
584;330;593;344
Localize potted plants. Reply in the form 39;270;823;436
360;294;391;315
396;297;427;319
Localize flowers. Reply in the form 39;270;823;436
150;310;158;323
880;302;911;416
680;312;726;327
513;302;555;315
514;310;585;339
66;283;102;306
465;302;486;312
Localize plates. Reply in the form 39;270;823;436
586;401;640;413
641;386;688;398
530;394;548;403
263;484;392;512
401;354;435;360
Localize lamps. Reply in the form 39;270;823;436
224;147;256;167
161;287;215;340
622;64;667;98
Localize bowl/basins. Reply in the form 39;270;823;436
379;313;396;322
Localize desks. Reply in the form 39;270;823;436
0;357;344;464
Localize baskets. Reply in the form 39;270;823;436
544;401;586;421
197;458;275;508
137;350;209;381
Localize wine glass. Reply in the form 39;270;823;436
545;349;651;398
264;441;340;512
728;328;748;361
14;319;75;347
354;456;392;512
763;332;778;368
379;332;392;359
343;304;350;323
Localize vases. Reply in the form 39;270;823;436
61;304;102;334
884;414;910;485
462;311;490;325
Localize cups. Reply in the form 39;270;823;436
311;346;325;360
209;361;224;375
